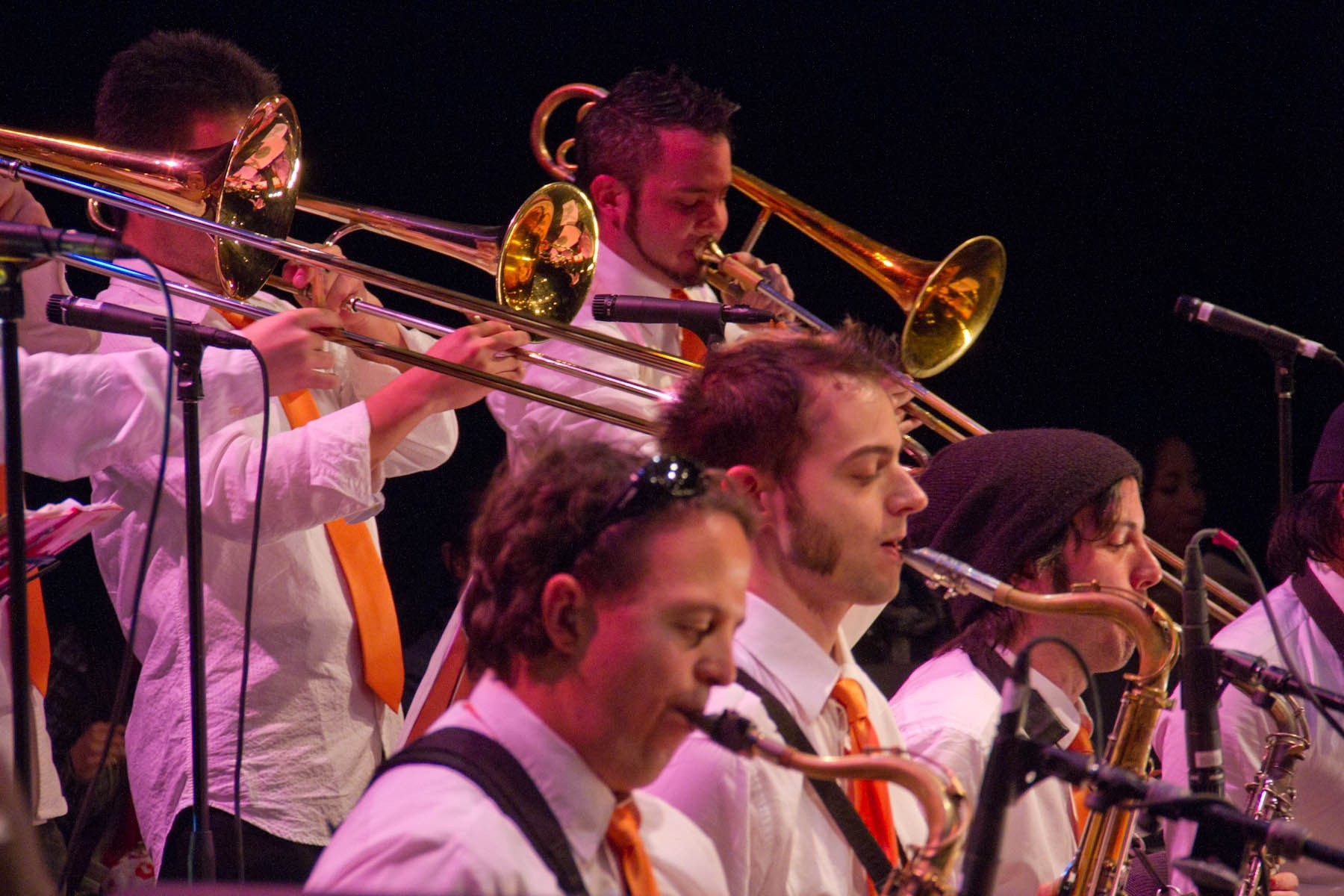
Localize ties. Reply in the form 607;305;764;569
191;278;404;717
0;467;51;699
608;803;662;896
829;677;900;895
1068;720;1098;836
670;288;708;366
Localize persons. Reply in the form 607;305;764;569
886;414;1299;896
0;32;531;896
303;435;761;896
1152;403;1344;896
486;70;930;462
634;336;1064;896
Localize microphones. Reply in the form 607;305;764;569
1174;542;1224;803
959;654;1029;896
594;293;776;328
1175;296;1335;364
0;219;138;261
47;294;251;350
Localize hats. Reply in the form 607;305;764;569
1309;401;1344;483
900;429;1143;632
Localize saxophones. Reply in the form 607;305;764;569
898;546;1180;896
1128;618;1312;896
682;706;968;896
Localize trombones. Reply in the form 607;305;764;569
531;83;1255;625
0;95;706;438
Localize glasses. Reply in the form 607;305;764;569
551;451;716;574
